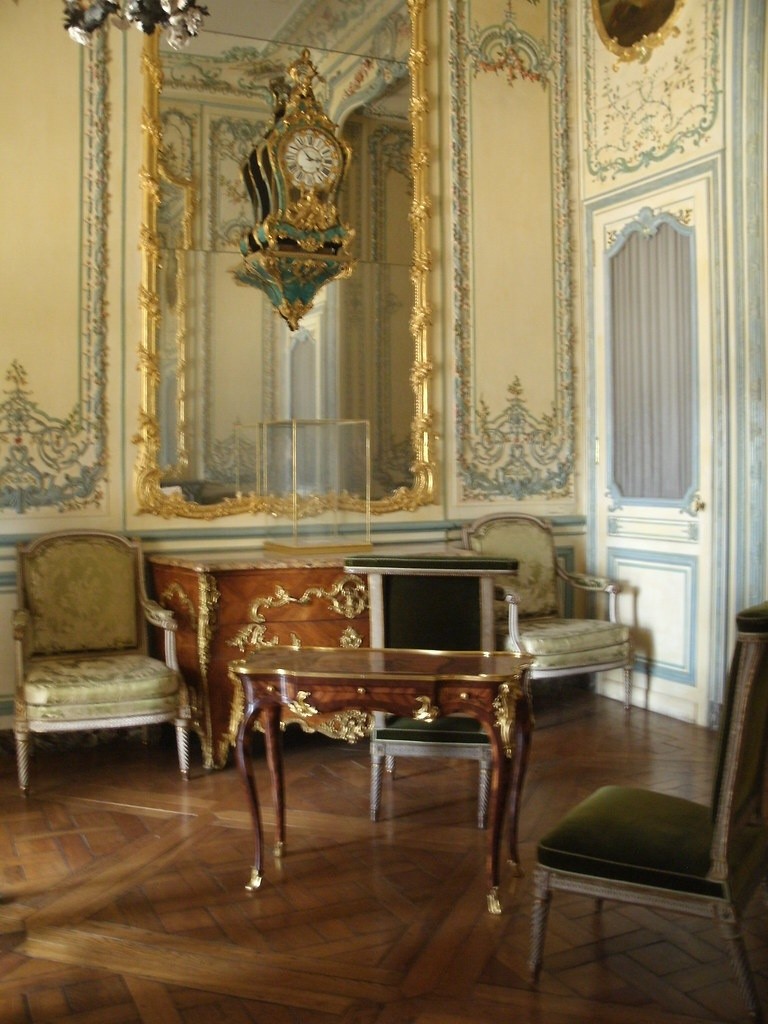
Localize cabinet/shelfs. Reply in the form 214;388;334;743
142;546;375;774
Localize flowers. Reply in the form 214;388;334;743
61;0;213;53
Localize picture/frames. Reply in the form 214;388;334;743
591;0;687;74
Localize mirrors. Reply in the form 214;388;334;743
131;0;446;522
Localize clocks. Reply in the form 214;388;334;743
227;47;360;332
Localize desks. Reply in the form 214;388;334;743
228;643;538;917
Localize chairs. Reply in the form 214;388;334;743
462;511;636;728
527;601;768;1024
10;528;193;798
342;553;528;831
